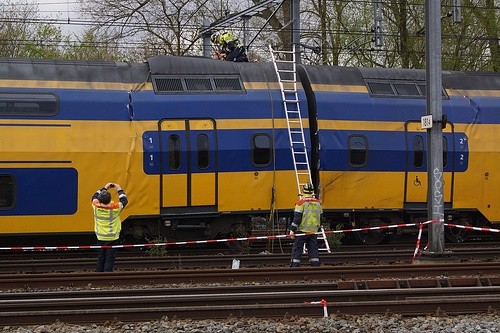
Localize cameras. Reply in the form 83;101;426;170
110;184;115;187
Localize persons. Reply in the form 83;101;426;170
288;182;324;268
210;31;249;63
91;182;129;273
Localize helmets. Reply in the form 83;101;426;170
290;230;295;239
302;183;314;194
210;33;218;45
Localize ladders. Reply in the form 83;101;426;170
269;45;331;253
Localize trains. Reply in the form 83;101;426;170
0;55;500;236
